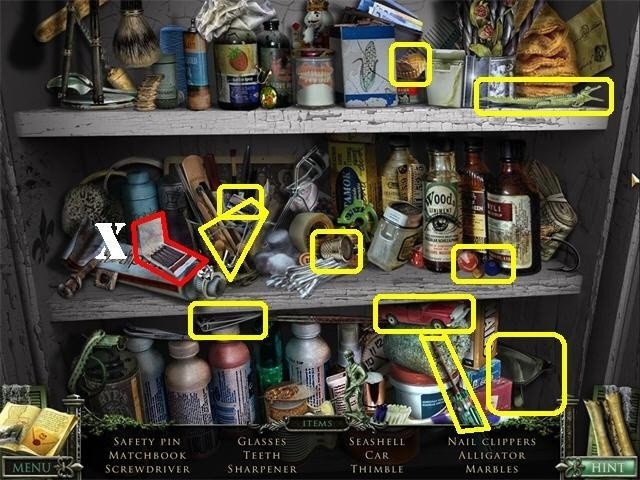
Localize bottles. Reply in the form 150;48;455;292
381;141;424;213
213;16;258;110
151;54;176;109
124;337;166;425
121;168;158;243
247;317;284;393
486;138;542;275
458;137;486;255
422;145;464;274
182;18;211;111
208;323;253;425
284;322;332;409
258;19;293;108
163;340;212;425
336;323;362;378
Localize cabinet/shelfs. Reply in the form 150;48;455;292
1;25;640;400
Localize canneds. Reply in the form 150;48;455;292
367;201;422;272
79;335;143;426
294;56;337;109
